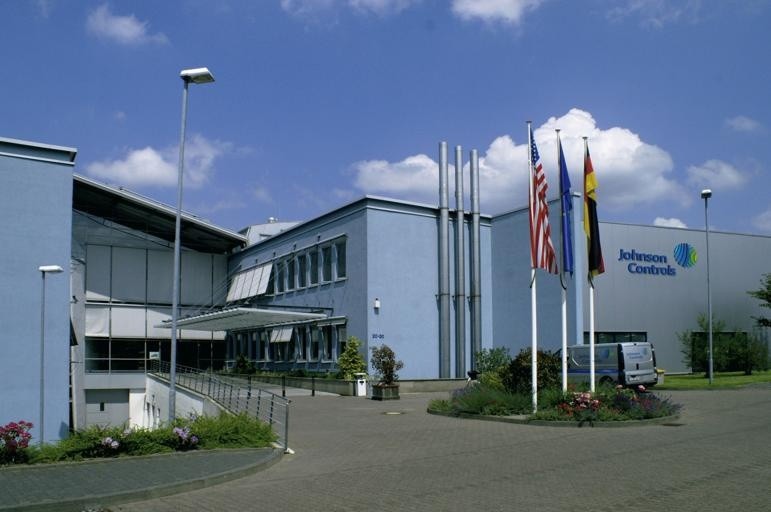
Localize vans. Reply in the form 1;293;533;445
553;342;659;389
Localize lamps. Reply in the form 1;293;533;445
374;298;380;309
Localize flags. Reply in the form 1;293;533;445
559;137;573;280
529;127;559;274
583;141;605;280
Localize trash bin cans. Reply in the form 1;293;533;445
224;360;236;377
352;373;367;397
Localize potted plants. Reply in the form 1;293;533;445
369;344;403;400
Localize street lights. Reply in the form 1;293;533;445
39;266;64;447
701;190;713;388
168;68;215;428
